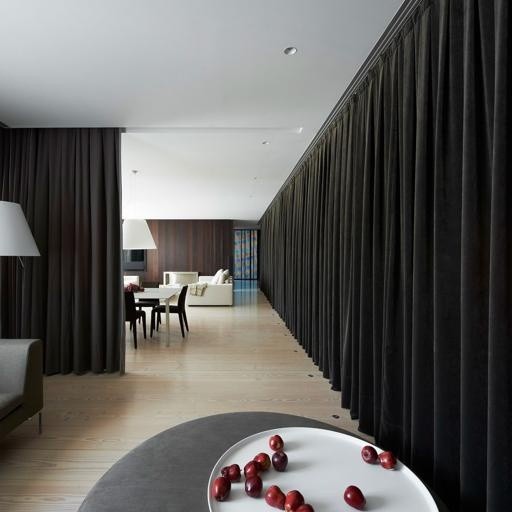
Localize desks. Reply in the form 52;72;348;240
77;412;376;512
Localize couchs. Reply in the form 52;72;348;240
189;274;234;306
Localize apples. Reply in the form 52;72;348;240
211;433;396;512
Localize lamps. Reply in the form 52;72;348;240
0;201;41;256
122;170;158;249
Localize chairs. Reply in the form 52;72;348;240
0;337;45;438
125;286;189;350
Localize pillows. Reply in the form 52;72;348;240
211;269;230;285
